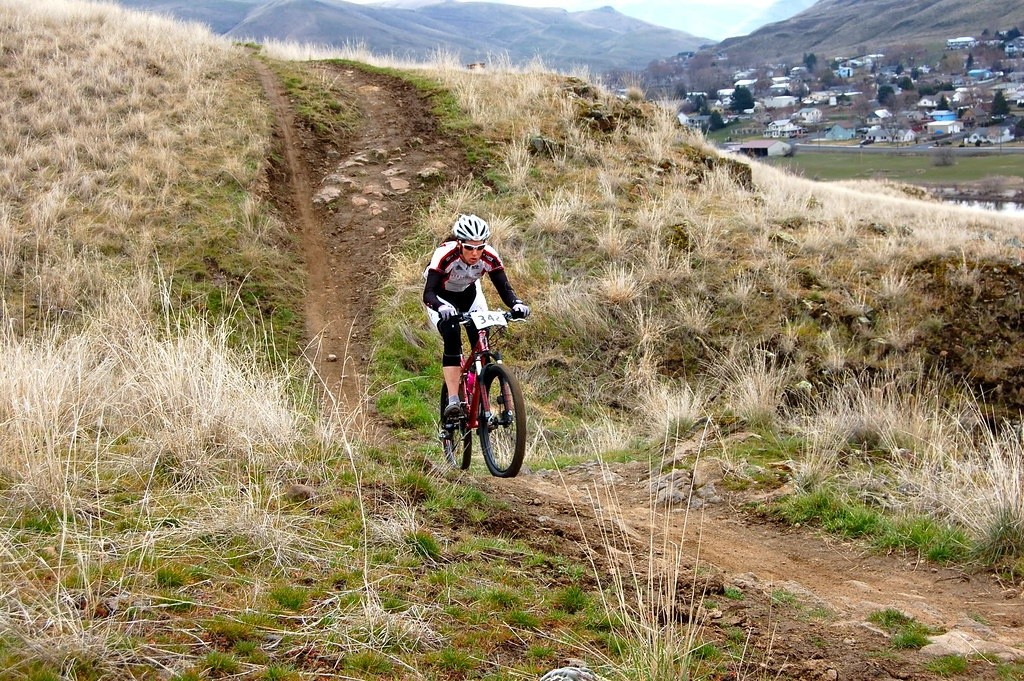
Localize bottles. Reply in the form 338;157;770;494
467;362;475;395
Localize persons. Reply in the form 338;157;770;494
422;214;530;418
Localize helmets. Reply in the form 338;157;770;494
452;214;489;241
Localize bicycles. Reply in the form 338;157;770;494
438;311;531;478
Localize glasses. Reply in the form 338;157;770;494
457;240;486;250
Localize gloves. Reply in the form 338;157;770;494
438;305;462;322
511;300;530;319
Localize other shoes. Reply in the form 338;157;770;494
476;421;496;435
444;401;460;416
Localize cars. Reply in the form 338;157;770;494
861;140;872;145
